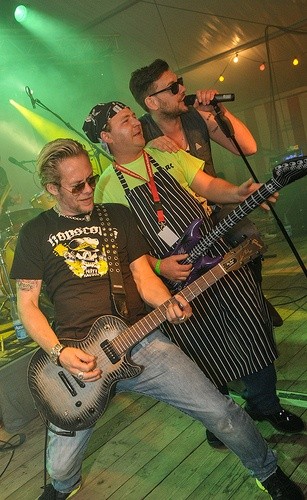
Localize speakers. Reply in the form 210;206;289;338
0;344;40;430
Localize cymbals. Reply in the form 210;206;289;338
0;208;44;231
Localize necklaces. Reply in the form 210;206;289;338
53;207;92;221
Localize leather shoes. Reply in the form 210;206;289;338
206;428;221;447
243;400;305;432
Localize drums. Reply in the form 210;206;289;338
29;192;58;213
0;223;24;298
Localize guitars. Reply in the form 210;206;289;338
27;235;268;431
161;149;307;297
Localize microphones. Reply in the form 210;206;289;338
27;86;36;108
184;93;235;106
9;157;28;170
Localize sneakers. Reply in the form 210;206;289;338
37;481;80;500
254;470;303;500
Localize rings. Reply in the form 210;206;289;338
180;316;184;320
78;372;83;379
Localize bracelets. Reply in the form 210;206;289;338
50;343;66;366
154;259;161;275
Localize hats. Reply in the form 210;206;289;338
82;102;128;143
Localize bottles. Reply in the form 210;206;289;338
10;298;32;345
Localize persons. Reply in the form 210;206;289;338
129;59;284;328
9;138;305;500
82;101;305;447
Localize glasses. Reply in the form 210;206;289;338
53;172;100;197
150;77;184;98
